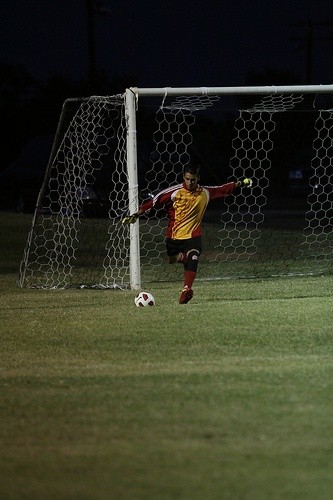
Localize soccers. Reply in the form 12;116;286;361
134;291;155;307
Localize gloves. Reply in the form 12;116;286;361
122;213;139;224
238;177;259;189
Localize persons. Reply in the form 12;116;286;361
120;164;253;304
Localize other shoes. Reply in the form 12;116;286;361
179;288;194;304
